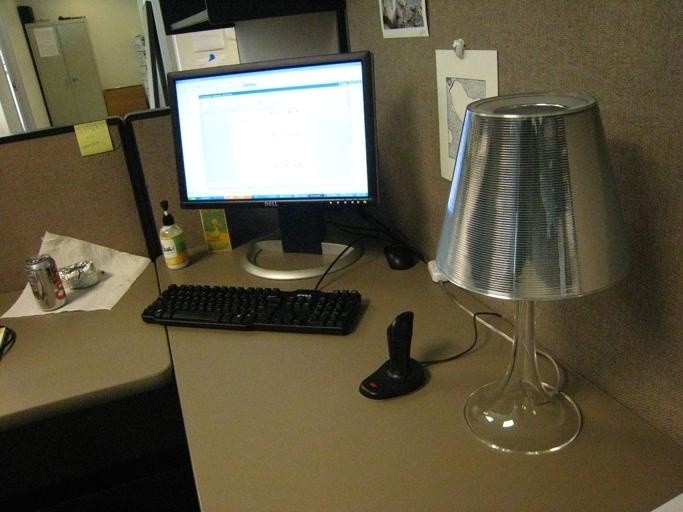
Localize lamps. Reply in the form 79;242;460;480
435;90;633;455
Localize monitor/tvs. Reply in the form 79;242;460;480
166;48;382;282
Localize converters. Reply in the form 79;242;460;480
427;259;451;283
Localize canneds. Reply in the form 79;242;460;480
24;253;67;312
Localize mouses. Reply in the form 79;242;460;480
383;243;412;270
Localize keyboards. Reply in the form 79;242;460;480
141;283;361;335
